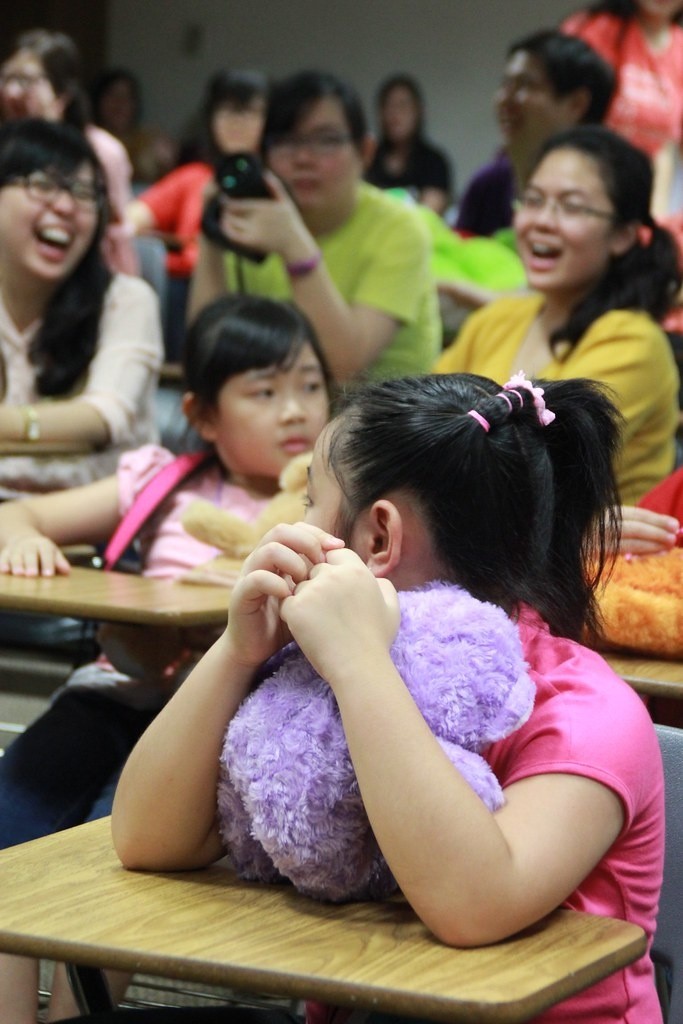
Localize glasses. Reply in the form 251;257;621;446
3;171;108;215
509;191;615;226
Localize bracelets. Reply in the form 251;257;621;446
287;252;319;277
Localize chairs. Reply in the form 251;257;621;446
643;720;683;1024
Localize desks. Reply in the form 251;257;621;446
1;355;681;1024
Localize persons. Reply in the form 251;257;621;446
0;0;683;554
111;374;662;1023
0;294;330;1023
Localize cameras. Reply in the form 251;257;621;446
200;153;275;265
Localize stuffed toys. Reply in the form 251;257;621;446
576;547;683;659
216;580;538;902
185;451;316;580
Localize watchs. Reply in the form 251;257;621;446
24;405;40;444
